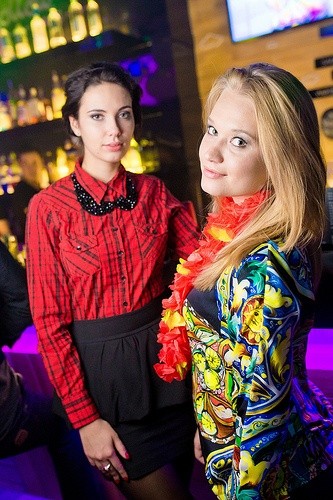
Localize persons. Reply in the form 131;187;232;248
7;145;42;243
0;240;32;441
154;63;333;500
25;62;203;500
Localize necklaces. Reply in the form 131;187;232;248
154;188;275;382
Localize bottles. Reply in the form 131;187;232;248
0;69;67;133
0;0;103;63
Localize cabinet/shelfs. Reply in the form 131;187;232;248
0;28;176;218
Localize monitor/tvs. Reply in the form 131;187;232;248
225;0;333;42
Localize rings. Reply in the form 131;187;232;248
104;464;110;470
